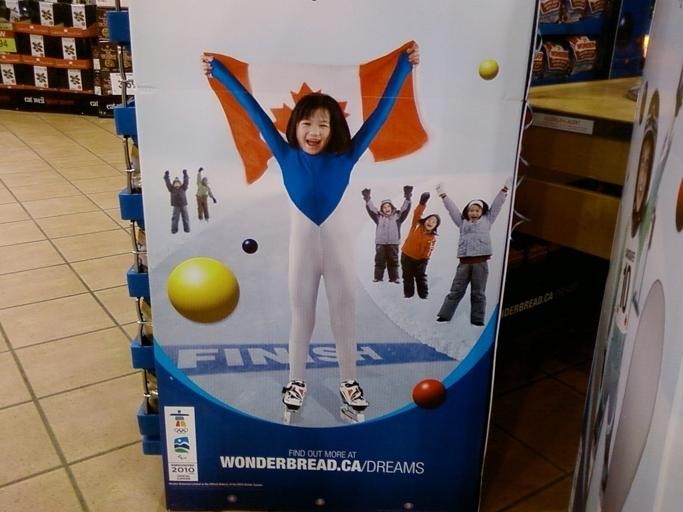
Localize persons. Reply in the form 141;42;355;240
163;169;191;233
201;44;420;410
401;191;441;298
196;167;216;221
433;176;514;326
361;184;413;284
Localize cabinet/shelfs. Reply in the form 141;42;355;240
531;0;624;86
0;0;104;112
102;5;170;459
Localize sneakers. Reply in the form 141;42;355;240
339;379;370;411
282;380;307;410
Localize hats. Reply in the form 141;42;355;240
173;177;181;187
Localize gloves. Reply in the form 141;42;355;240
504;176;514;189
183;170;187;174
434;183;445;197
362;188;371;201
165;170;169;175
403;185;413;198
420;192;430;204
199;167;203;171
213;198;216;203
